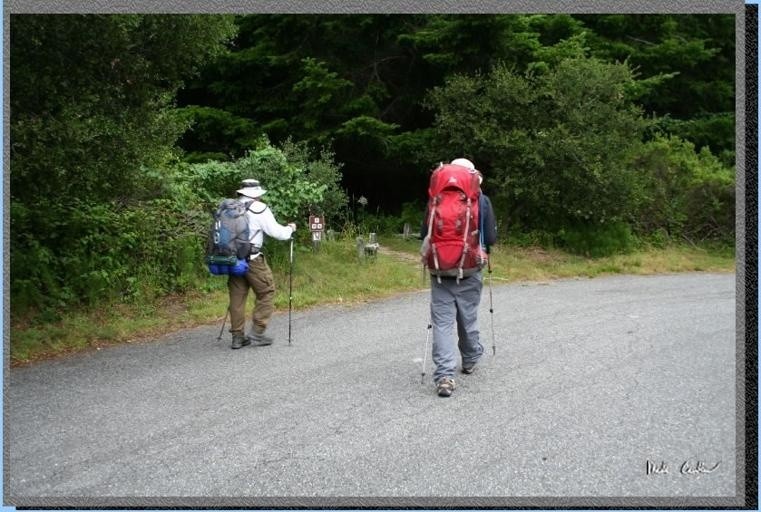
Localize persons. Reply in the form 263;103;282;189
420;158;497;396
225;179;297;349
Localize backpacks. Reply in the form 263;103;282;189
205;200;248;275
422;164;487;277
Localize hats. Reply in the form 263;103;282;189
237;179;267;198
450;159;483;186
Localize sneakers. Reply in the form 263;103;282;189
250;335;272;346
436;377;455;397
461;362;477;374
231;335;250;349
